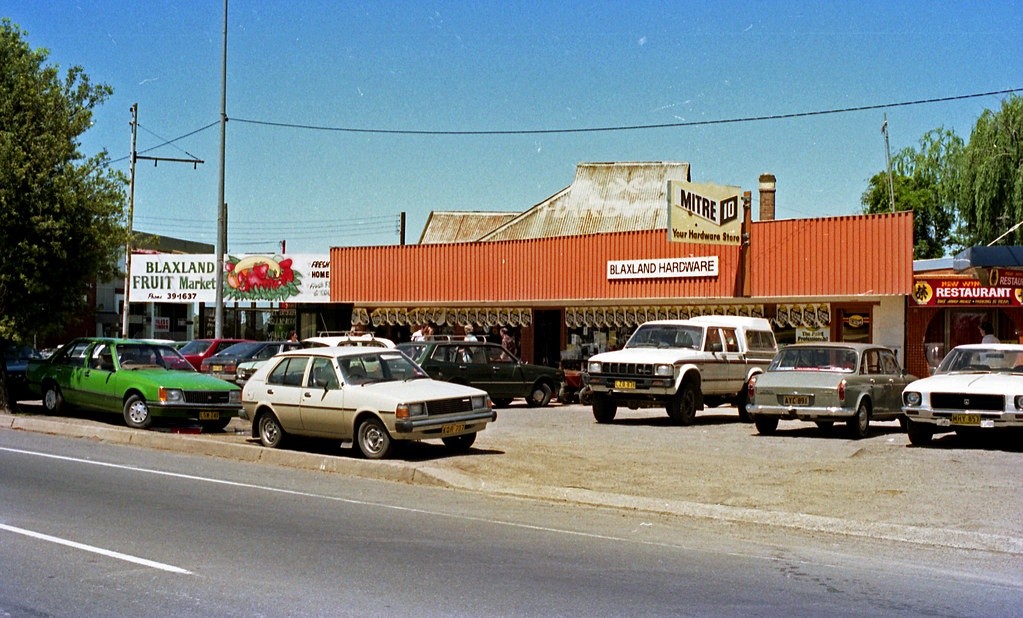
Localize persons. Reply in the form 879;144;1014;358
978;322;1004;365
411;323;434;361
287;331;299;351
499;328;517;359
458;325;479;362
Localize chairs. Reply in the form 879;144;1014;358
314;367;337;388
472;348;485;364
120;353;134;362
350;366;366;375
96;355;114;371
969;365;991;371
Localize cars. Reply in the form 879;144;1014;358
901;344;1022;445
744;337;920;434
40;339;208;362
200;340;305;383
375;340;564;409
238;347;497;460
24;338;242;432
6;345;44;399
235;336;397;390
150;340;258;371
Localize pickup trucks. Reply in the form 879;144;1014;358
579;313;779;424
